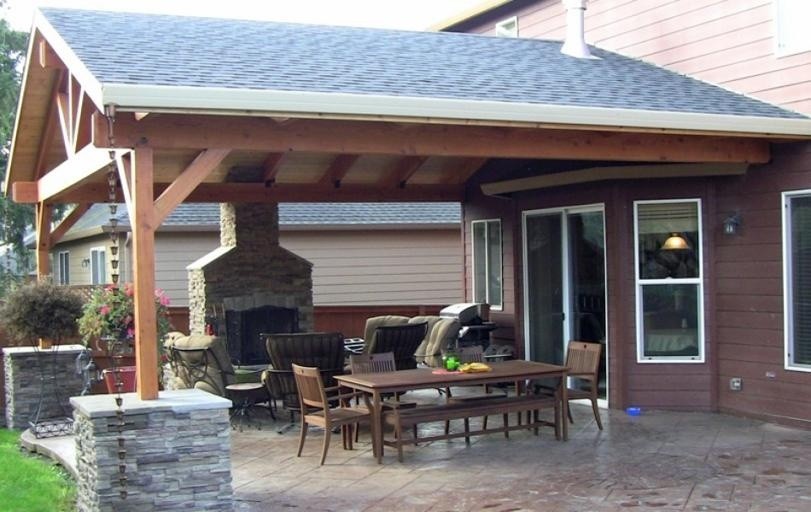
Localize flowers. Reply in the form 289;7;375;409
76;279;172;370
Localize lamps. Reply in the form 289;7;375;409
723;215;739;235
662;232;690;254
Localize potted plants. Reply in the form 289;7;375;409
101;348;137;392
0;273;83;348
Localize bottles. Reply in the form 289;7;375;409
442;347;460;372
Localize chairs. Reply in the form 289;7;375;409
291;363;377;467
532;339;604;442
658;312;690;330
349;352;418;448
161;315;461;426
439;346;511;445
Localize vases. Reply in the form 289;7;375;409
88;334;133;349
94;349;135;370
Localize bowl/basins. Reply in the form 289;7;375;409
627;406;640;416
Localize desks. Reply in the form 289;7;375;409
644;329;698;356
332;359;572;464
226;383;265;431
